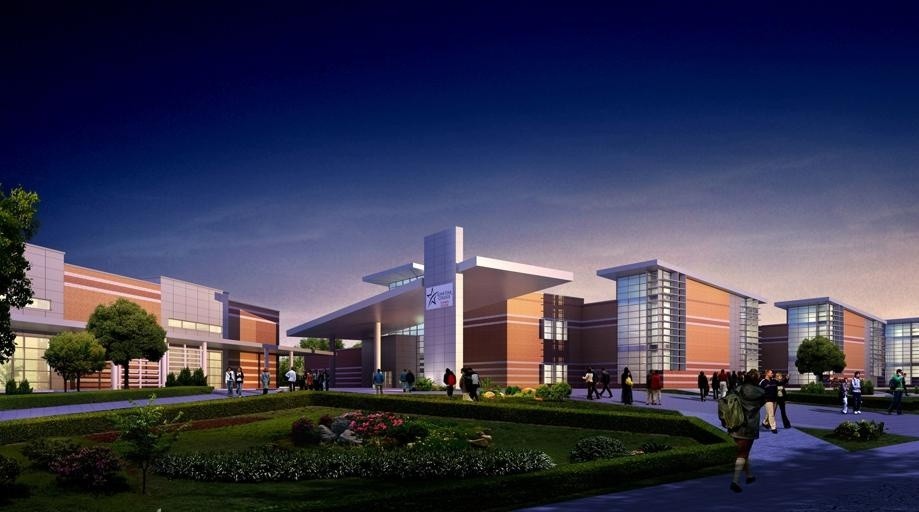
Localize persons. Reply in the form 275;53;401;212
262;368;270;395
400;369;408;393
581;368;663;407
285;367;330;391
234;368;244;399
444;366;480;402
887;369;905;416
850;371;862;415
840;377;849;414
225;367;236;398
373;369;384;395
404;370;415;393
697;368;791;492
902;373;910;396
